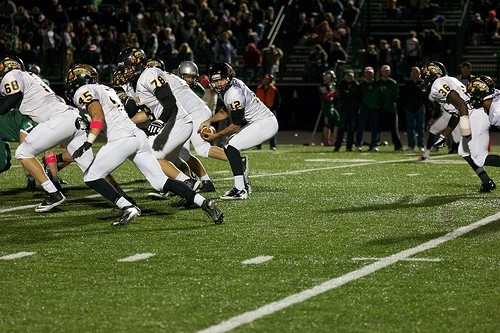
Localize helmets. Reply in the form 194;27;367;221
65;63;99;95
108;46;166;87
419;61;447;94
466;74;496;109
208;61;236;95
178;61;199;82
24;63;41;75
0;55;25;78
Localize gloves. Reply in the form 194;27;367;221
25;174;36;193
52;175;63;189
75;116;91;130
147;120;164;135
70;132;96;160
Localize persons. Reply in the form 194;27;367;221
0;0;500;225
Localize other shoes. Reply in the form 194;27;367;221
322;138;451;159
477;178;496;192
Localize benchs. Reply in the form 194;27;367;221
190;0;500;87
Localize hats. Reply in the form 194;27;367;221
322;65;390;83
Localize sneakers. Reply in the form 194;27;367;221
241;154;250;177
146;177;215;206
219;182;252;200
34;189;66;213
112;204;141;226
200;198;225;225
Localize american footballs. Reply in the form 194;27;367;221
200;125;216;139
